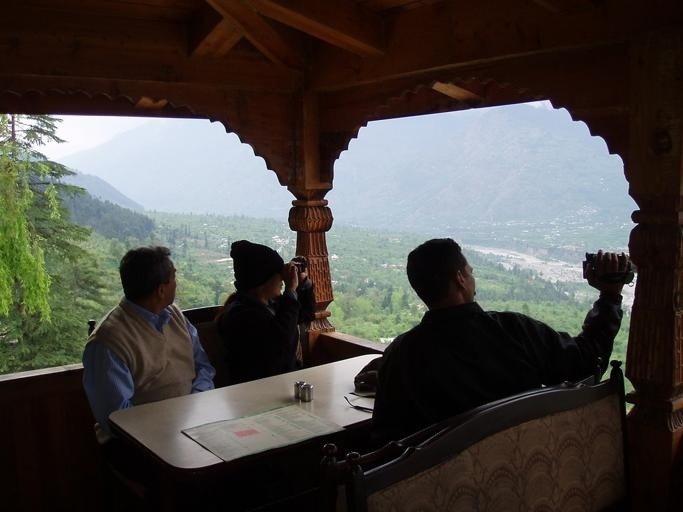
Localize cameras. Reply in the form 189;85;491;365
293;258;309;273
584;252;635;285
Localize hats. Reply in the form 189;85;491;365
230;240;284;290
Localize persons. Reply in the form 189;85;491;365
82;245;217;440
207;240;316;387
360;240;632;470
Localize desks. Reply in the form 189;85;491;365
107;353;383;510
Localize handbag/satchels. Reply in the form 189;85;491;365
353;358;383;397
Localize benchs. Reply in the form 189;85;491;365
86;302;313;501
316;355;635;512
